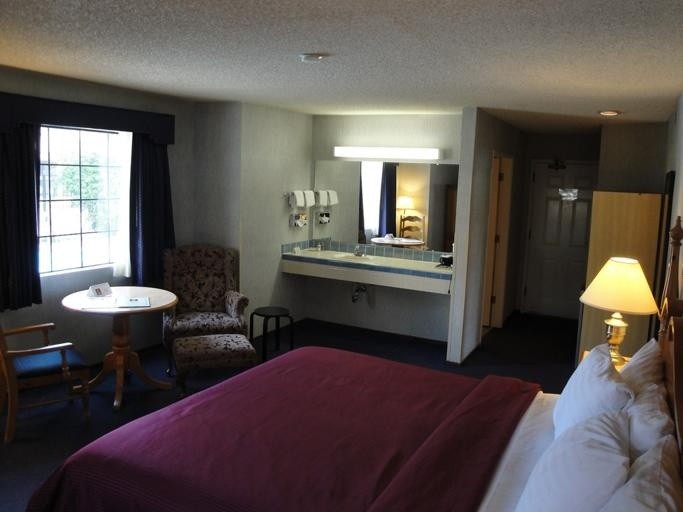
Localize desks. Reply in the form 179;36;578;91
62;286;179;409
370;237;425;248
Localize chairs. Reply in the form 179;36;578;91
399;215;426;249
162;245;257;373
1;322;90;444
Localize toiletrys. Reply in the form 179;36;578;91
316;211;330;224
289;213;309;227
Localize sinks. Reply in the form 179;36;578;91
335;253;370;263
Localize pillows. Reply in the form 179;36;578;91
513;338;681;512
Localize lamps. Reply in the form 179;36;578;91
396;196;414;237
579;257;660;367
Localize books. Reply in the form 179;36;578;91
114;295;151;309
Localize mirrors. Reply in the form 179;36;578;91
312;160;459;266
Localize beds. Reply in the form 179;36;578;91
78;315;683;511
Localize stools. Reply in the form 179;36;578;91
250;307;295;364
174;333;255;395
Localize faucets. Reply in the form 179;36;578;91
354;244;375;256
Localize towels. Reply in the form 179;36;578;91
315;189;338;207
289;191;315;208
